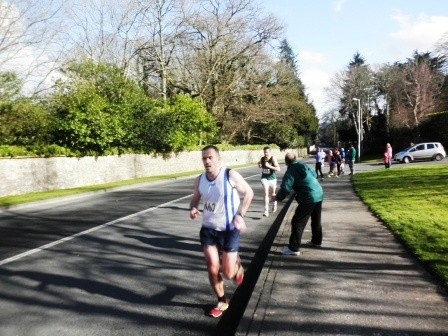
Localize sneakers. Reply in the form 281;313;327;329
282;249;299;256
307;240;322;248
234;255;244;286
209;298;229;317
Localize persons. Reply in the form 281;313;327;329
189;144;256;317
314;145;356;181
272;152;324;256
384;143;393;168
258;146;281;217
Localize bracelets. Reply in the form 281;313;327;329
237;212;242;217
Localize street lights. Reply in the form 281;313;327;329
352;98;360;163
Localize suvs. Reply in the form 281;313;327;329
395;142;446;164
